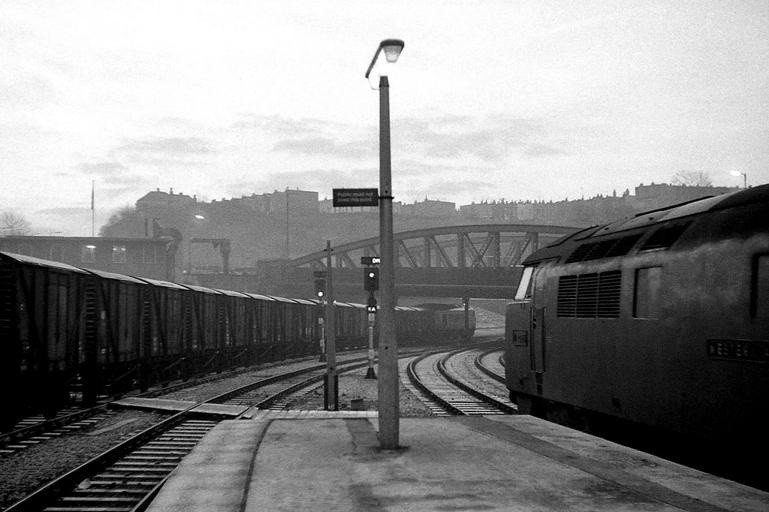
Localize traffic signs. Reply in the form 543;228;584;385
333;188;378;207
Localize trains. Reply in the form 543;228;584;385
502;179;768;473
0;250;476;428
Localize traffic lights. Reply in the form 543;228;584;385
363;266;379;291
367;298;378;314
315;281;325;299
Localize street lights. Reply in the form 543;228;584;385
364;38;406;453
729;169;747;190
